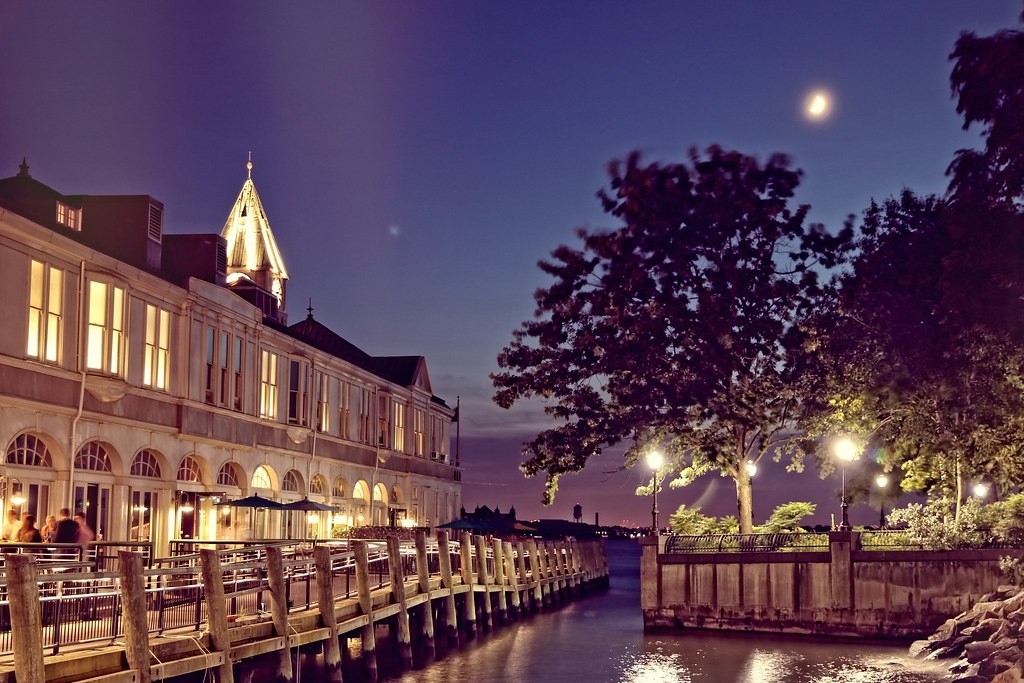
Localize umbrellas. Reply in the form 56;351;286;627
436;516;539;537
269;497;346;549
213;493;291;541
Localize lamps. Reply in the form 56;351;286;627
172;490;194;512
9;477;27;505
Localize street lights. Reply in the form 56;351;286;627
747;460;757;520
835;439;854;531
646;450;662;535
876;474;888;530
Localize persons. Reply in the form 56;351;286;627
0;509;94;594
